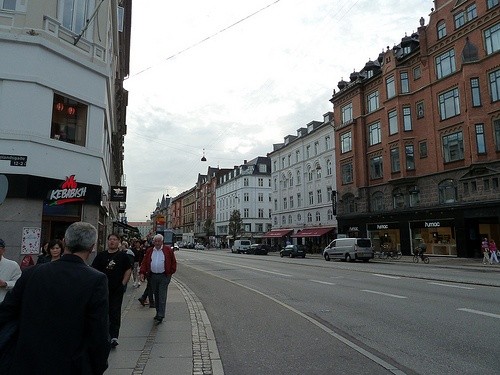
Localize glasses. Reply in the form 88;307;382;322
108;238;119;241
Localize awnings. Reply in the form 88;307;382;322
262;229;293;238
291;227;335;238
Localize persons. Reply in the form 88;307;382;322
119;232;159;287
481;237;500;265
0;238;23;303
138;234;177;326
35;238;66;264
91;231;132;347
0;221;110;375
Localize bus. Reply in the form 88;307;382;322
155;230;177;253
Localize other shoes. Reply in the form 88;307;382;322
149;305;156;308
138;298;145;306
110;338;119;348
154;315;163;323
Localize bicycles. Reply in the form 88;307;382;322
378;247;403;260
413;248;429;264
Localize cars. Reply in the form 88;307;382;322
279;245;306;258
250;243;269;255
173;240;205;251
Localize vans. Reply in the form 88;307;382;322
231;240;252;254
322;238;374;263
